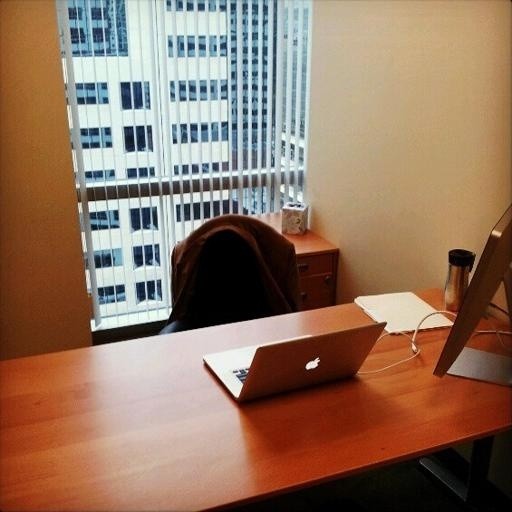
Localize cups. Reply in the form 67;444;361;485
445;249;476;311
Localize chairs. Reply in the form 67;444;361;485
159;215;299;337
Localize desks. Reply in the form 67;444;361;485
1;287;511;511
254;211;339;311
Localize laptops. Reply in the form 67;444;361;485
202;322;387;402
